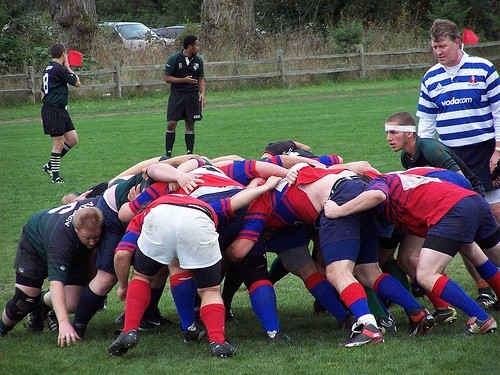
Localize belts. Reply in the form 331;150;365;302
328;175;360;199
164;201;214;220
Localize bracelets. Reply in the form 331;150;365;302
495;147;500;152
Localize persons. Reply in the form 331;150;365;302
386;112;496;310
164;35;205;157
415;19;500;226
0;194;104;348
48;139;500;362
41;43;80;184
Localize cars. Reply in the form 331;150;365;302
129;25;202;39
99;21;176;53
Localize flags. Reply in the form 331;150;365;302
462;30;478;44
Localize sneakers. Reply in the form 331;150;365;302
475;293;497;310
108;328;140;356
410;276;426;298
52;176;65;184
462;313;497;335
375;315;397;334
115;313;125;322
138;320;161;330
266;329;291;342
46;310;60;334
345;323;386;348
210;340;238;358
183;324;206;344
339;313;359;331
403;306;436;337
225;309;233;322
313;299;325;313
98;295;108;311
431;306;458;326
42;163;53;178
27;303;44;331
72;323;86;339
